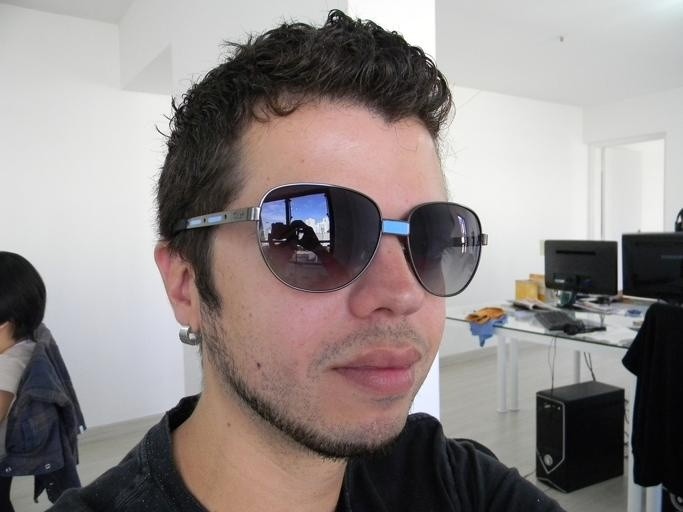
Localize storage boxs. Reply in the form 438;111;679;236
513;274;551;303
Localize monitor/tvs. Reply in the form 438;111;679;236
621;233;682;302
544;240;617;311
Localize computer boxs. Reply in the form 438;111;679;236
536;382;624;493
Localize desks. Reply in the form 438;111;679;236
443;300;681;512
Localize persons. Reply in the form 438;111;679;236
33;9;565;510
0;251;88;512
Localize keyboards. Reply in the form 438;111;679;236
536;310;573;331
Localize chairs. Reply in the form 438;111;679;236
0;322;88;512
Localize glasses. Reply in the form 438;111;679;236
159;182;489;298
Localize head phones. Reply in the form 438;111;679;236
564;320;584;335
